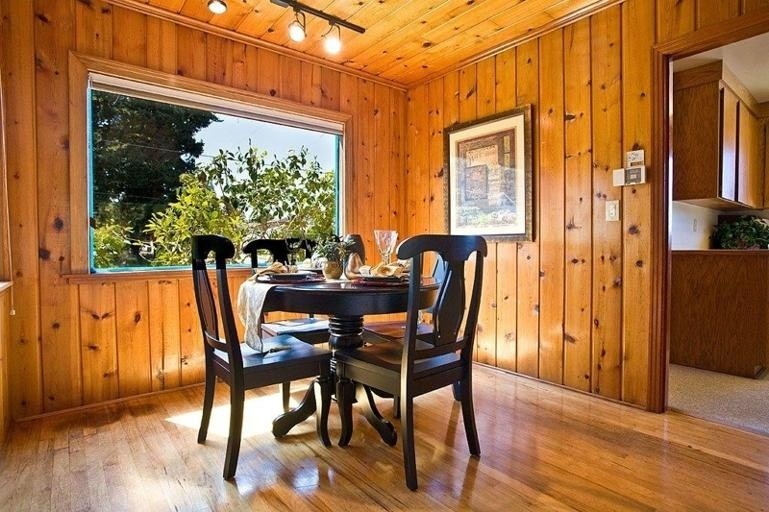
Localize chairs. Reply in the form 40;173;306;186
188;233;341;480
418;254;451;317
240;234;345;411
334;235;487;491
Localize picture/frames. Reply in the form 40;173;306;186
442;104;535;242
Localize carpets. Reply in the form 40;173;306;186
667;363;768;439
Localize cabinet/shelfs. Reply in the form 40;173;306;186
673;59;768;211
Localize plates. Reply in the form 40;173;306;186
362;274;403;282
255;275;325;284
351;278;408;286
264;270;318;280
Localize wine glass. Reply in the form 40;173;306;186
374;229;397;267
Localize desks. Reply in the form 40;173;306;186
243;269;439;448
